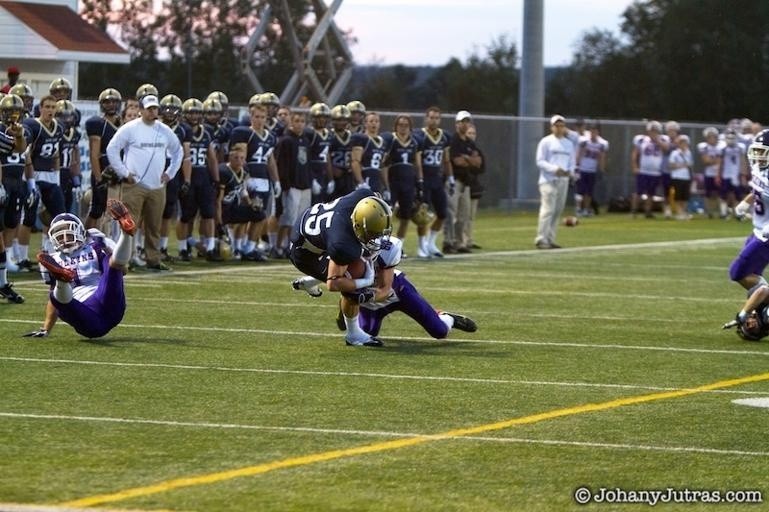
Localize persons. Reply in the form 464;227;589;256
631;114;768;340
565;116;609;214
534;114;576;249
0;67;487;346
575;124;606;216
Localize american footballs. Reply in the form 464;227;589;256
562;215;578;226
344;259;367;279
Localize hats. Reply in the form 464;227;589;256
646;118;768;169
551;114;568;126
455;109;472;123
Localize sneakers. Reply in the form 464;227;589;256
344;333;386;348
1;260;39;303
107;199;136;232
534;240;561;248
401;242;483;259
336;297;348;330
131;246;291;270
576;206;594;217
643;210;691;222
37;253;77;280
292;276;322;298
434;305;477;333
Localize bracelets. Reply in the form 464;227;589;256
576;163;580;166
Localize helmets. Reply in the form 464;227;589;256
97;86;229;125
351;196;394;261
415;204;439;229
48;213;87;253
248;93;280;112
210;239;232;261
737;310;765;339
309;100;367;122
0;78;74;121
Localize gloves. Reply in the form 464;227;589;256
274;181;281;198
342;289;375;304
312;179;321;194
327;180;336;195
356;261;377;288
27;188;42;209
23;327;48;338
